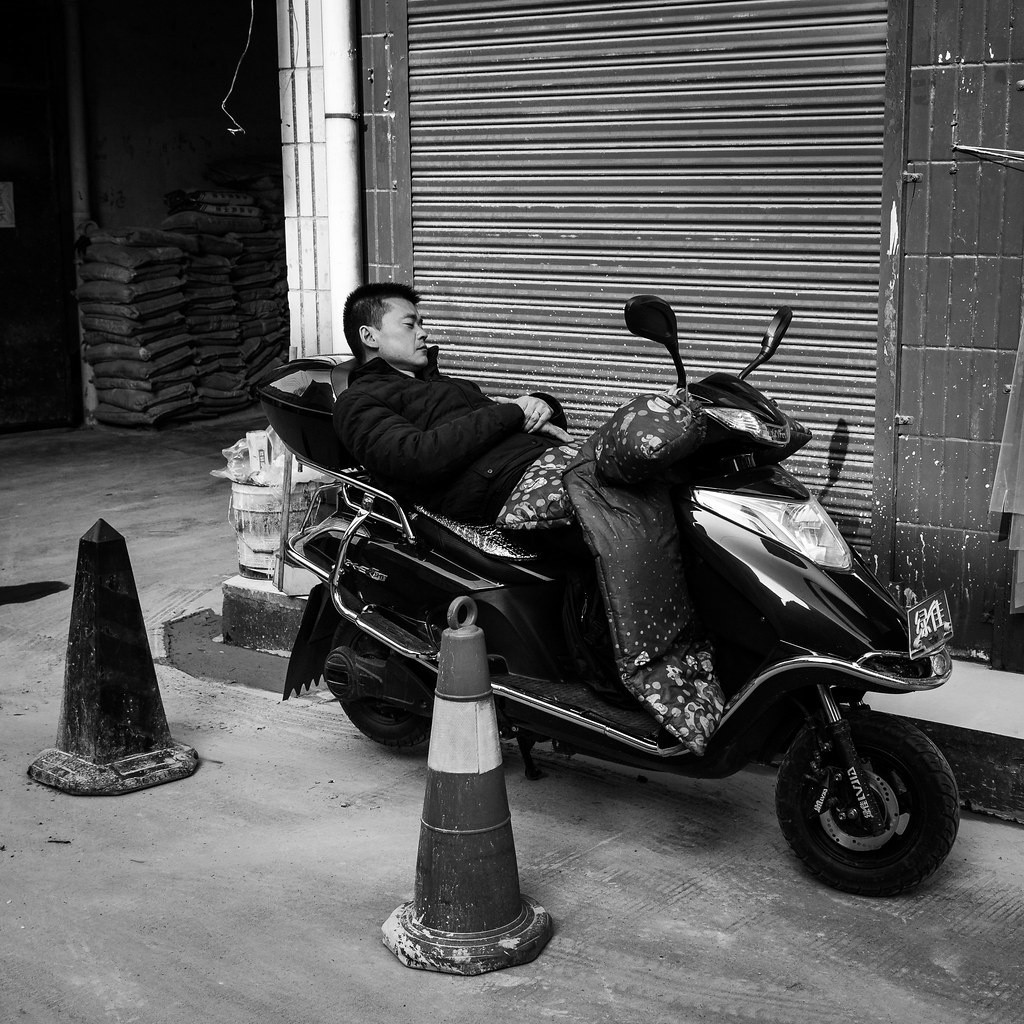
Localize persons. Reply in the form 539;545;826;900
334;283;626;704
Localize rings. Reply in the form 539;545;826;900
534;410;541;417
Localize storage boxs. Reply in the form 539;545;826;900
249;354;360;472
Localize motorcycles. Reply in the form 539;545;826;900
245;291;962;899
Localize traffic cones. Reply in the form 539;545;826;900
377;595;557;978
26;514;201;797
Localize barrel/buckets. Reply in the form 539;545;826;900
226;480;320;580
